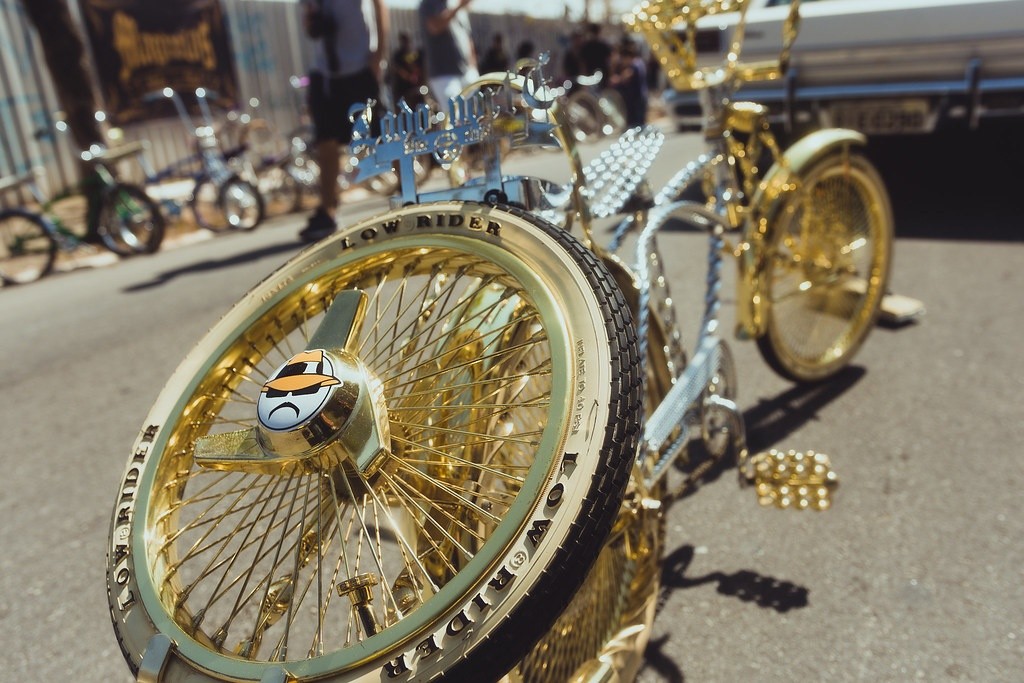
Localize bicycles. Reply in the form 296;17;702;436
104;5;895;683
0;58;628;290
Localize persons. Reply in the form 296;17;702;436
309;18;652;143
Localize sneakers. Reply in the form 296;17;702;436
298;214;337;239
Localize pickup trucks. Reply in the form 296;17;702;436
664;0;1024;189
